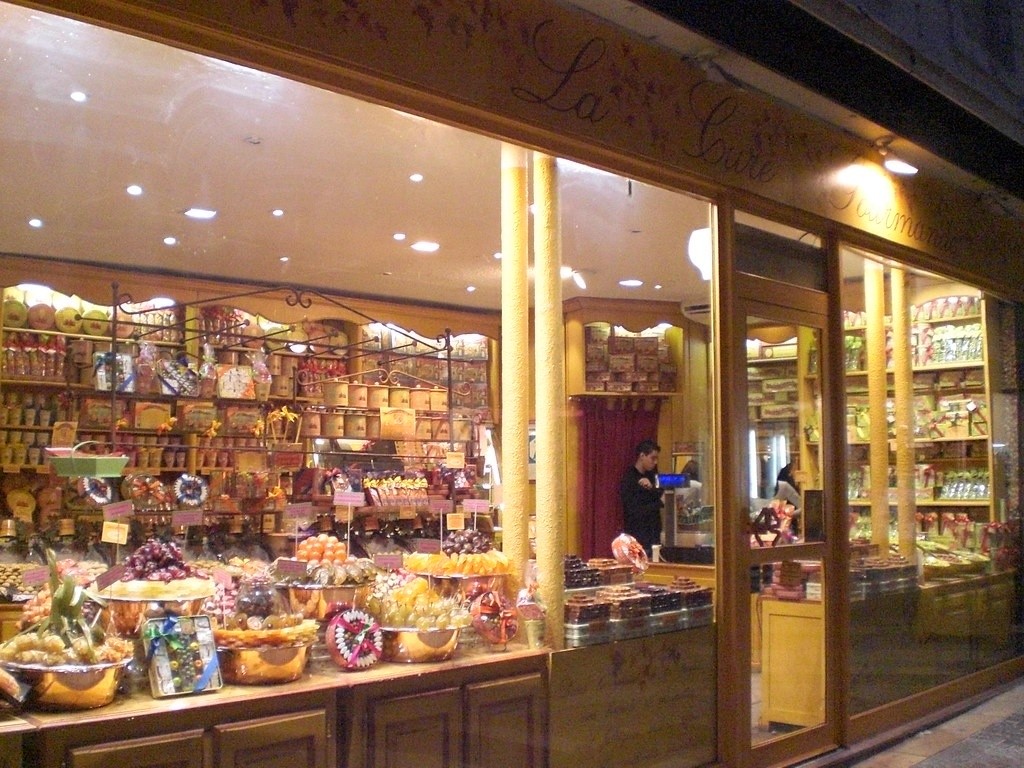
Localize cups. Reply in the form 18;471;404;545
523;620;545;650
650;544;662;563
80;431;257;468
0;389;79;464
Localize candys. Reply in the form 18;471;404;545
122;526;506;685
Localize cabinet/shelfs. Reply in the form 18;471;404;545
312;642;551;768
1;326;478;517
760;567;1017;733
798;278;1000;507
26;667;338;768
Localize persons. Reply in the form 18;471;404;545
773;462;800;535
675;461;703;506
620;440;664;557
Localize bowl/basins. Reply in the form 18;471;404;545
274;580;379;622
410;569;512;600
212;635;321;685
377;620;474;662
0;640;132;711
88;584;218;635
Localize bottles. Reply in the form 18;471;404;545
51;518;85;565
0;519;24;565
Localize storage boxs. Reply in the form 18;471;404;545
76;477;116;507
144;615;226;698
41;485;67;521
92;351;135;393
587;325;676;393
176;399;219;434
561;533;716;648
222;407;261;437
746;363;800;419
158;360;202;398
173;474;209;507
4;488;38;516
471;592;519;642
214;364;257;401
322;611;384;672
79;398;125;428
130;399;171;431
